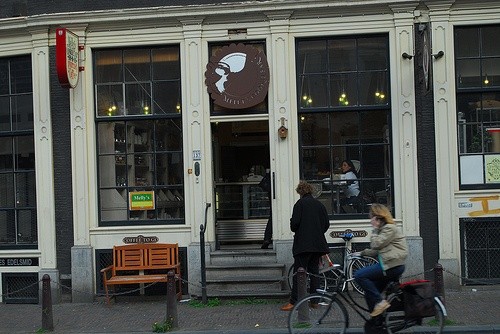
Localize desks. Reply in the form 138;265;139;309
310;181;349;213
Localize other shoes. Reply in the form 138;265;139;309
261;239;271;248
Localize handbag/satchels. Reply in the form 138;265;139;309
318;254;334;274
403;281;438;320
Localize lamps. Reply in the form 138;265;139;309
108;99;118;114
401;49;414;61
434;51;444;61
337;77;349;106
302;78;314;104
175;89;180;113
483;73;491;87
370;74;387;101
143;94;149;115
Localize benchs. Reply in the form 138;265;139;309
101;242;182;305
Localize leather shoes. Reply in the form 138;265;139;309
280;304;297;311
310;303;321;309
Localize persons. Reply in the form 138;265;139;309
324;160;361;214
280;182;330;310
259;171;272;249
355;204;408;317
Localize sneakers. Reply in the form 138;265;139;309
368;301;390;317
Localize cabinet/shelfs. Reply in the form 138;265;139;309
97;121;184;219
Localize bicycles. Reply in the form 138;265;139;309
286;232;383;304
285;252;448;334
